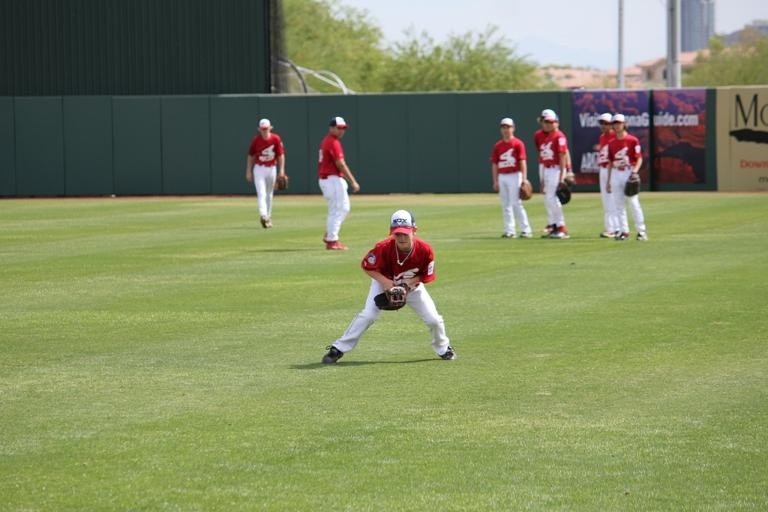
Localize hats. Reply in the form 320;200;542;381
499;108;626;126
329;116;347;128
259;118;271;130
388;210;416;235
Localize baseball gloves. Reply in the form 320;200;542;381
275;176;288;190
556;181;571;205
520;182;532;199
564;172;576;187
374;282;411;309
624;175;641;197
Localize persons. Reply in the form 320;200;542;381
318;117;360;251
596;113;623;239
489;109;575;239
246;119;288;229
606;113;647;242
323;209;457;364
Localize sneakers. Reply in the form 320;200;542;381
439;346;455;359
326;241;345;249
502;224;647;240
321;346;343;363
261;216;271;227
